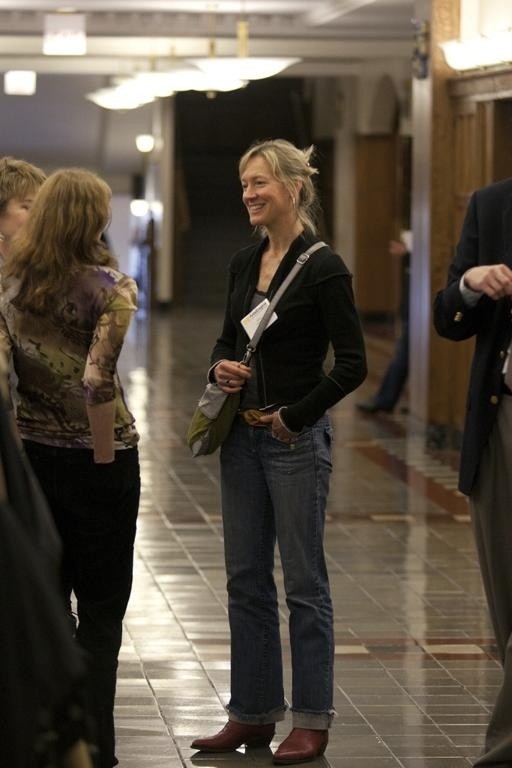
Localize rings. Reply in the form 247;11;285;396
226;379;231;387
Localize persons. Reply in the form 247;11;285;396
186;136;370;763
357;236;412;414
0;157;49;266
0;167;144;765
430;179;511;768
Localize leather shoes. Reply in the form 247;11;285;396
191;721;275;752
356;397;393;412
272;728;329;764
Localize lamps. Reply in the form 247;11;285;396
88;17;302;109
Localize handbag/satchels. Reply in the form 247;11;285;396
187;384;241;455
